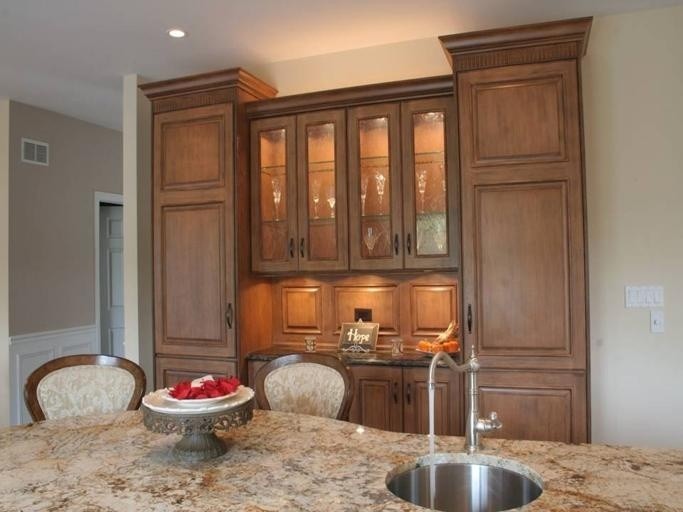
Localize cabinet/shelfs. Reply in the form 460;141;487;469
437;14;594;444
136;67;279;395
249;75;457;275
247;361;463;435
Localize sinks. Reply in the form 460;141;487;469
385;452;545;512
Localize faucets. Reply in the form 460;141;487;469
427;351;483;390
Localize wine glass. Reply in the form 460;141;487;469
417;162;428;215
311;174;336;219
361;168;386;217
269;176;282;221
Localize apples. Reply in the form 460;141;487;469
418;340;458;353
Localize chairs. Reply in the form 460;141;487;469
23;354;146;422
255;353;356;421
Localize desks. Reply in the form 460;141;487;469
0;409;683;512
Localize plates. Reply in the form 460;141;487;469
162;385;240;405
415;349;460;359
